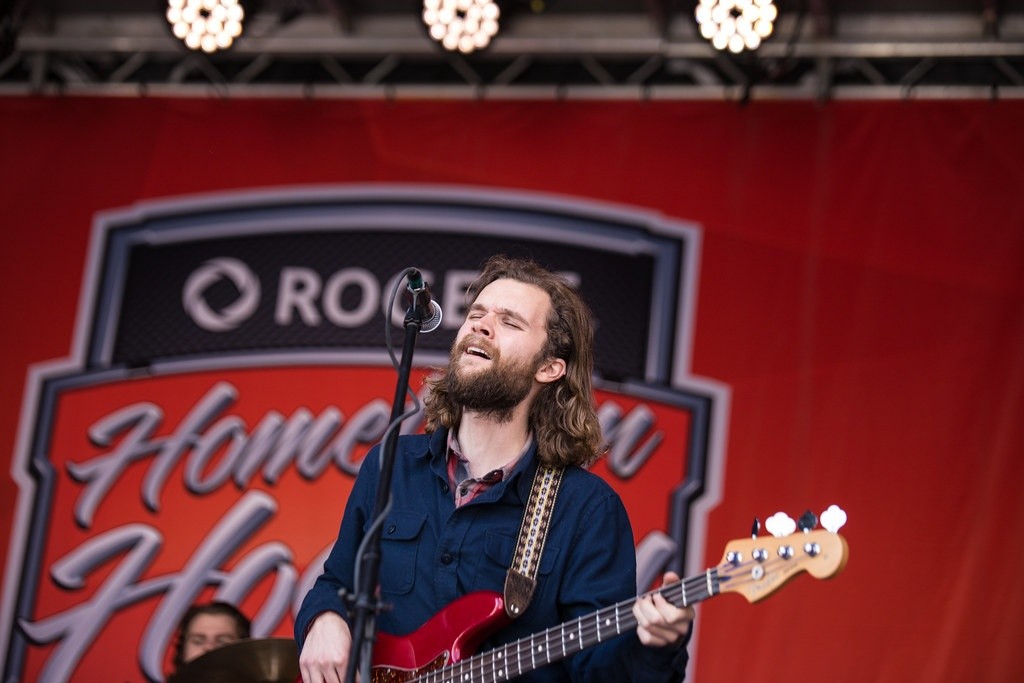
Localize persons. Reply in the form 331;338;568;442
174;602;251;665
295;258;694;683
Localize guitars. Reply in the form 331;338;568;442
157;502;849;683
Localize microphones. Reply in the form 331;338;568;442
405;267;442;332
